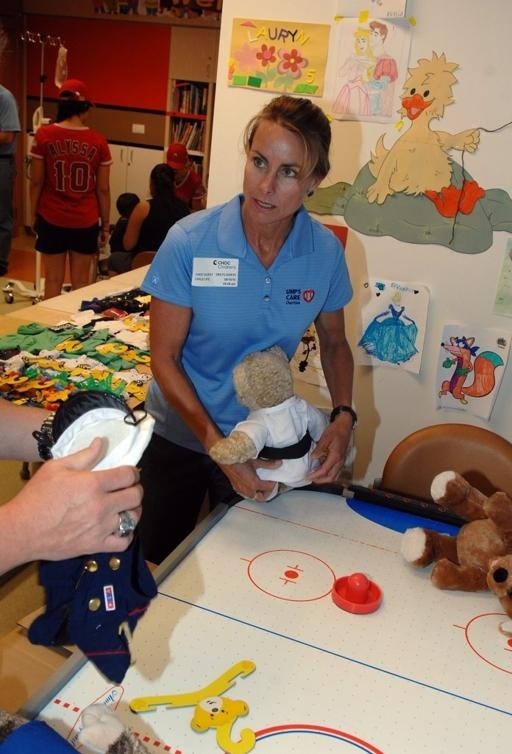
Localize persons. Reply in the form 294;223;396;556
109;191;141;252
1;384;145;579
108;162;191;273
0;84;23;276
29;78;115;300
138;92;355;566
165;142;207;214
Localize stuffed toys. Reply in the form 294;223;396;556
24;390;156;683
208;343;359;502
399;468;512;623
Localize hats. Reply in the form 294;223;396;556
57;80;90;101
166;143;189;171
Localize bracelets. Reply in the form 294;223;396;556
329;405;357;431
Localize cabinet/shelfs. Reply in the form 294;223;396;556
167;26;213;217
104;143;162;230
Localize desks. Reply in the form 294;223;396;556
1;264;161;462
12;475;512;753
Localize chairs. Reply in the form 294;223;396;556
381;422;511;507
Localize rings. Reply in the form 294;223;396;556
118;511;137;537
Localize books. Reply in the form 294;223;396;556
171;83;210;177
